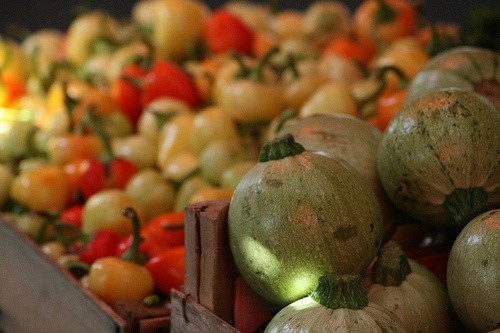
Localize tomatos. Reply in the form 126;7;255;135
0;0;470;305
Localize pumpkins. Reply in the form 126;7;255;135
228;88;499;333
